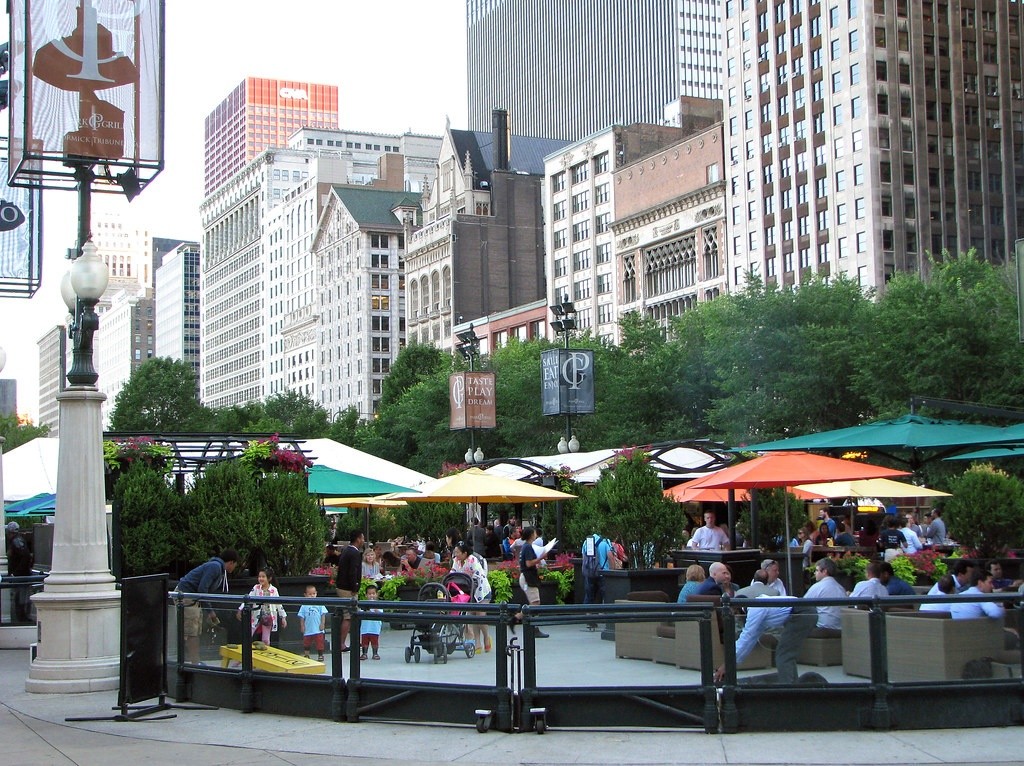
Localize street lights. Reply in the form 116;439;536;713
455;321;484;464
24;231;124;694
549;293;579;549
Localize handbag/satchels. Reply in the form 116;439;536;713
524;569;541;587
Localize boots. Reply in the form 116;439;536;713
534;627;550;638
508;616;519;634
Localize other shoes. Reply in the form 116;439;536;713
342;647;350;652
360;654;368;660
475;648;481;654
318;655;324;661
456;643;473;650
372;654;380;660
484;635;492;653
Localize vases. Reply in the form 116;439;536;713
509;580;558;605
542;476;561;486
387;584;428;629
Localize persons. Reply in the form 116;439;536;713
674;561;917;664
792;508;857;568
324;533;493;659
581;529;657;626
918;562;1024;648
880;508;946;562
297;587;327;662
681;513;749;550
174;550;239;663
237;568;287;645
467;516;550;638
715;596;819;682
6;523;33;621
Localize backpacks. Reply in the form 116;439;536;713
17;540;34;567
581;536;607;578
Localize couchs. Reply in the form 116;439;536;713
614;590;1024;682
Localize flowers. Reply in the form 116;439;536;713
804;544;1018;586
309;564;338;590
357;551;578;621
606;444;654;471
438;461;468;478
538;463;573;493
843;450;868;461
256;432;315;477
113;435;156;465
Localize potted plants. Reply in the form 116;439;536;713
941;462;1024;587
112;457;326;653
735;482;807;597
570;446;693;641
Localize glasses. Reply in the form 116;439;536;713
765;561;775;570
797;531;804;534
459;540;464;547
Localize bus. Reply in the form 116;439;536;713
826;505;886;547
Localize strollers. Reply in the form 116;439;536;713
405;572;476;664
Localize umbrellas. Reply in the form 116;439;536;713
686;450;914;595
373;468;579;552
661;474;825;547
305;461;422;543
721;412;1024;522
795;479;952;533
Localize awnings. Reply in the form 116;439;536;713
6;493;55;517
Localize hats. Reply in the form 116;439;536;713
4;522;20;533
